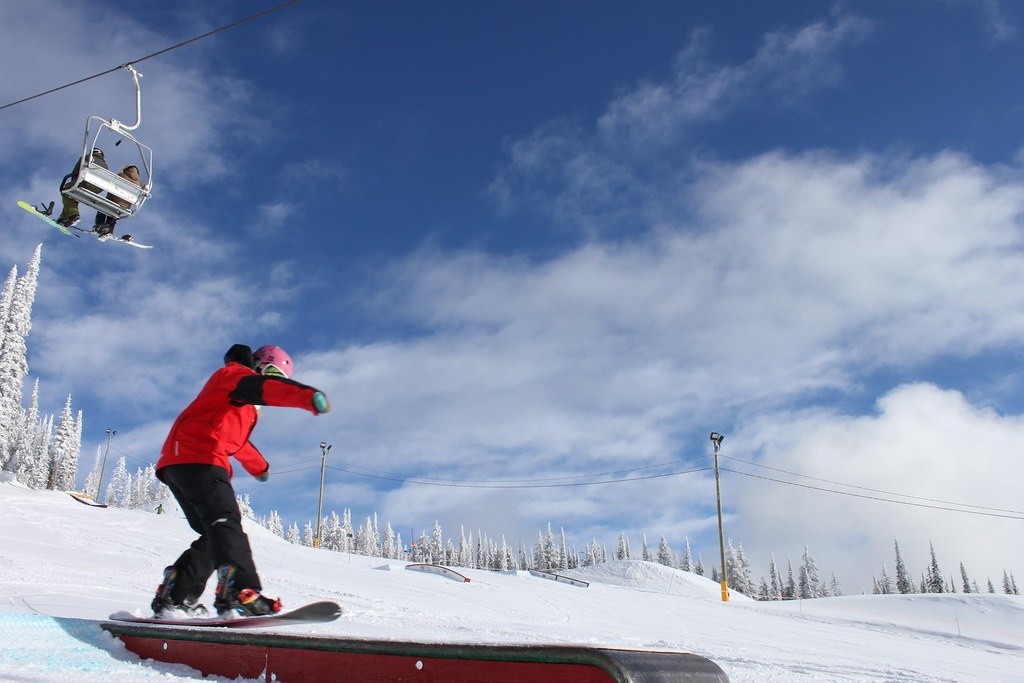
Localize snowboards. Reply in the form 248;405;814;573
16;199;82;239
70;225;155;250
108;601;344;629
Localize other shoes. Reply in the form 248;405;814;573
93;224;113;235
56;214;80;226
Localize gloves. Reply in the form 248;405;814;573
313;391;331;414
256;470;269;482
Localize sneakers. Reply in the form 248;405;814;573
151;566;210;618
213;562;282;616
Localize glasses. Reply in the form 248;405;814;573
261;364;289;379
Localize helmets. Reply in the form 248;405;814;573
252;345;294;379
90;148;103;157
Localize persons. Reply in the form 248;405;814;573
150;345;329;620
93;165;142;236
53;148;109;228
